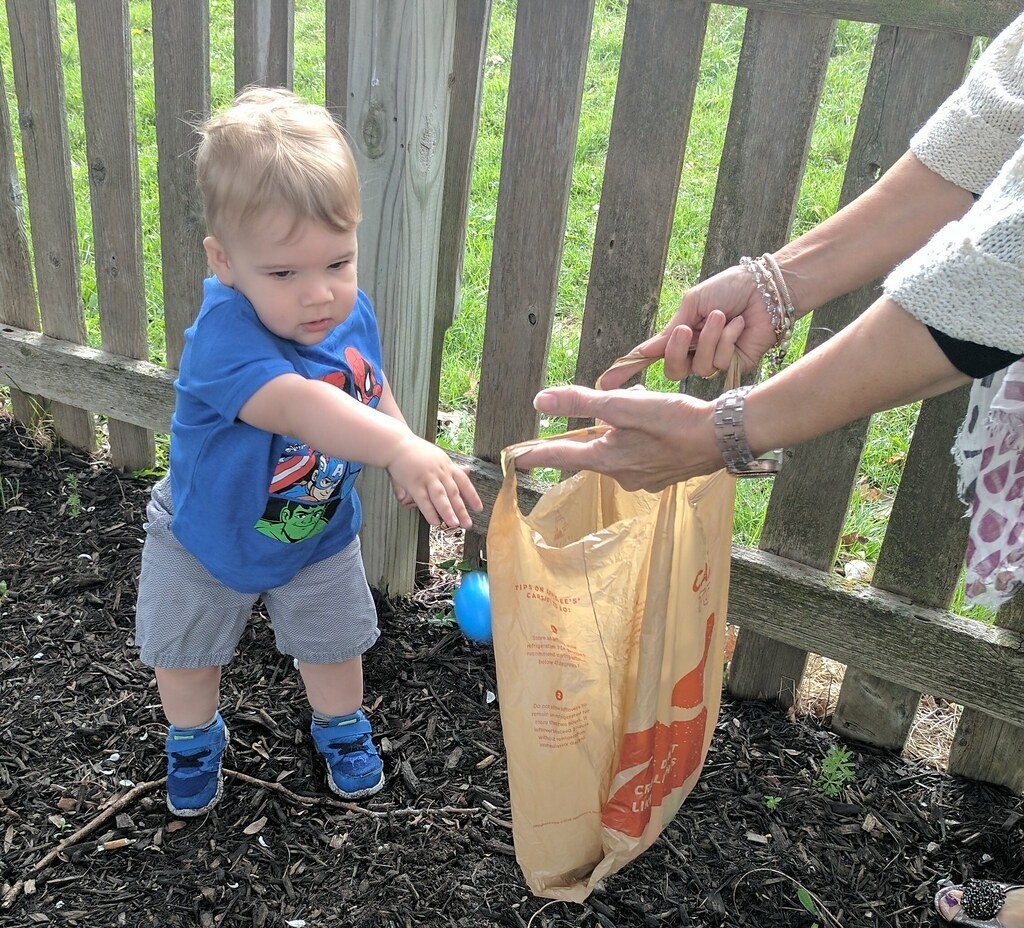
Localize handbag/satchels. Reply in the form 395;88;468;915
485;346;740;905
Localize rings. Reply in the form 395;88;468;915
702;368;721;380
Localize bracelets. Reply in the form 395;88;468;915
738;251;796;368
713;384;784;478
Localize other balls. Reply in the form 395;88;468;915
455;566;496;646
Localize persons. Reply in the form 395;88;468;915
135;83;487;816
502;11;1024;928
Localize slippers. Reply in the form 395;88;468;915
935;878;1024;928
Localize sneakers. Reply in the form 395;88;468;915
310;710;385;799
165;713;230;816
